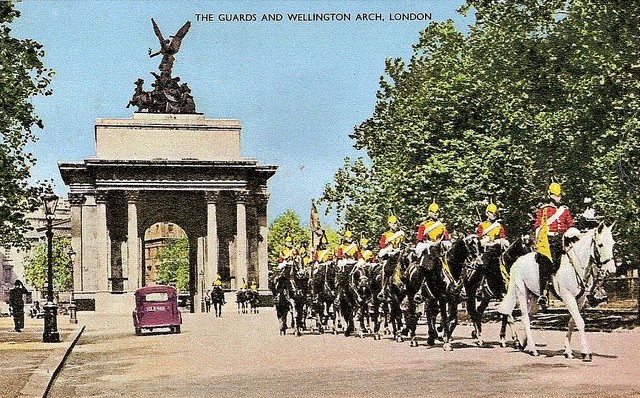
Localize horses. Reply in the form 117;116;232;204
211;287;224;319
496;221;620;362
274;220;535;352
235;290;260;315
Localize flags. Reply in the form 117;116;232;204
309;202;327;248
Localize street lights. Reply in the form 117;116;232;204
67;246;78;324
41;194;61;342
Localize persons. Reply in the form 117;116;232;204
273;232;297;301
334;227;357;308
459;197;506;302
248;278;257;292
414;195;449;304
9;280;30;333
299;243;310;266
357;235;374;263
210;274;227;305
204;291;211;312
535;177;574;306
240;277;247;292
314;229;334;263
377;211;405;301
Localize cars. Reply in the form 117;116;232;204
132;285;182;333
